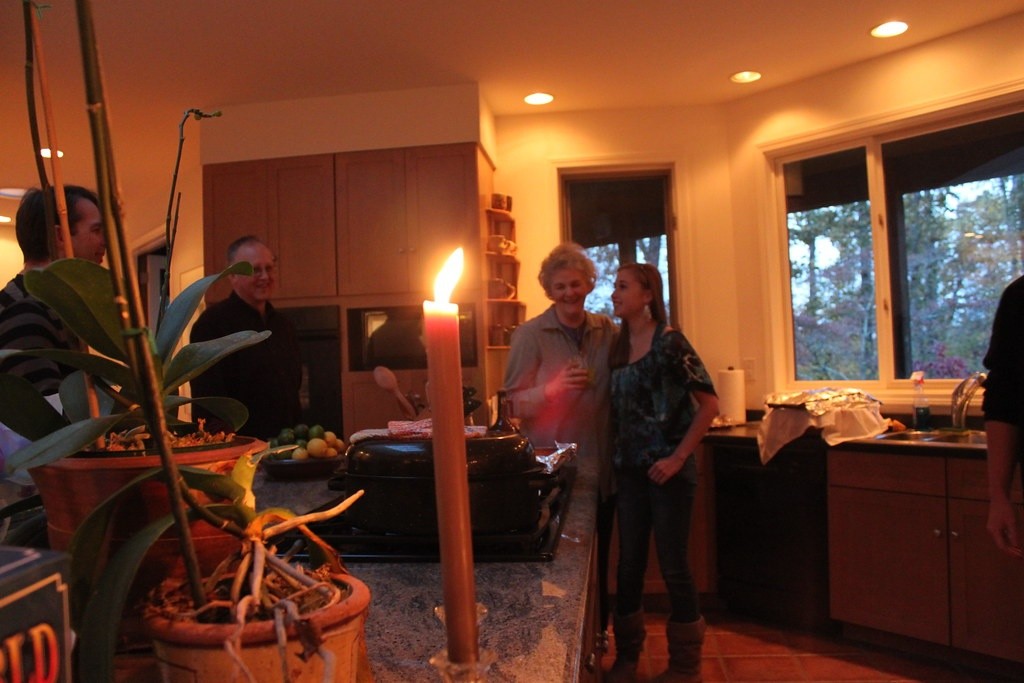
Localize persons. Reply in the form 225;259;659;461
978;271;1024;553
184;234;303;457
497;240;623;649
1;180;112;428
600;263;722;683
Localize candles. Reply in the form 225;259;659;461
424;247;480;683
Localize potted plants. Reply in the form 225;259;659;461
0;254;271;655
66;444;371;683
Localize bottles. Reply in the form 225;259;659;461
473;390;537;473
510;326;516;345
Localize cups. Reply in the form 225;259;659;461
490;326;504;346
491;193;508;210
566;352;590;391
489;235;509;252
489;279;505;299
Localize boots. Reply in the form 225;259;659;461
651;614;706;683
604;607;647;683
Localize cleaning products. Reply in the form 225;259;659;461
911;370;930;430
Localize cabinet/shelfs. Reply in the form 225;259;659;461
195;143;526;451
831;453;1024;662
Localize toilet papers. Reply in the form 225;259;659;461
717;368;747;423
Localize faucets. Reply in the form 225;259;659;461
951;372;986;428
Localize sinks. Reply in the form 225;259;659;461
925;431;987;445
874;431;952;443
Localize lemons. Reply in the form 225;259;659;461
268;425;344;460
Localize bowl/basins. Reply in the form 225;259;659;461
465;426;487;437
260;457;345;481
